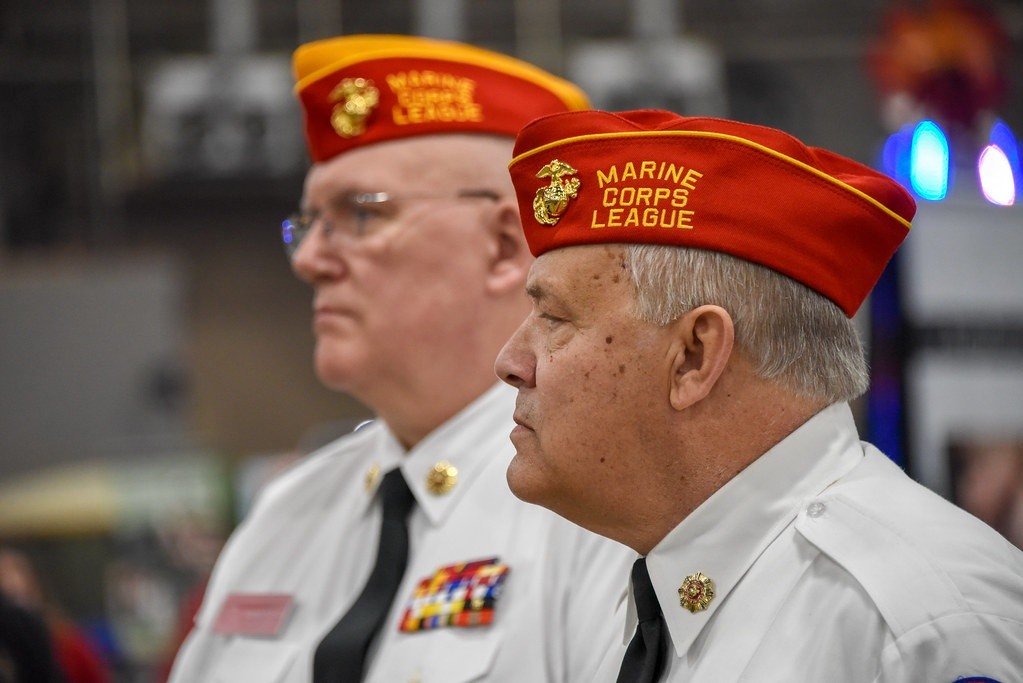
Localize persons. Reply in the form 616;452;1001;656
166;34;642;683
870;15;1023;551
493;110;1023;683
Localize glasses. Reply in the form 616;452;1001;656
280;189;505;259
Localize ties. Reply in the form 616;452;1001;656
614;558;669;683
311;468;422;683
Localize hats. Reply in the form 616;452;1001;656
292;36;595;164
506;108;918;319
